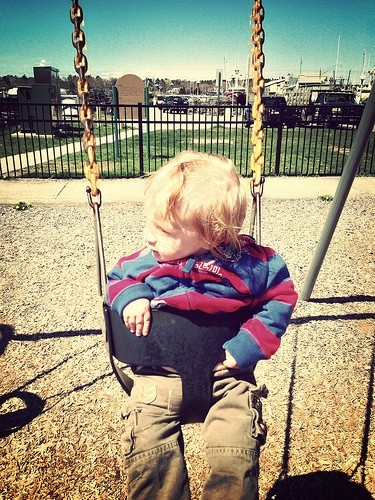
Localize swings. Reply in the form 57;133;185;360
70;0;266;425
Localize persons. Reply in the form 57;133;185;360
104;150;298;500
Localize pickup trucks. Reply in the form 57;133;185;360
302;90;364;128
161;96;190;112
245;97;302;128
